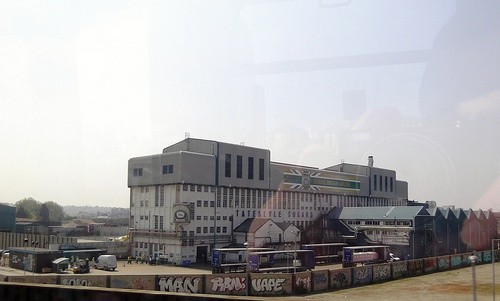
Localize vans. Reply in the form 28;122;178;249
389;253;400;262
94;255;117;271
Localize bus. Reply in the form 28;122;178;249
75;226;87;232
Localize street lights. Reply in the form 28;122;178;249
103;212;107;242
23;238;40;275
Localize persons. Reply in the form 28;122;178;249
135;255;138;264
0;251;3;260
141;255;144;264
127;255;132;264
90;256;95;266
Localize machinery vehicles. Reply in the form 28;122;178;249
72;258;90;274
